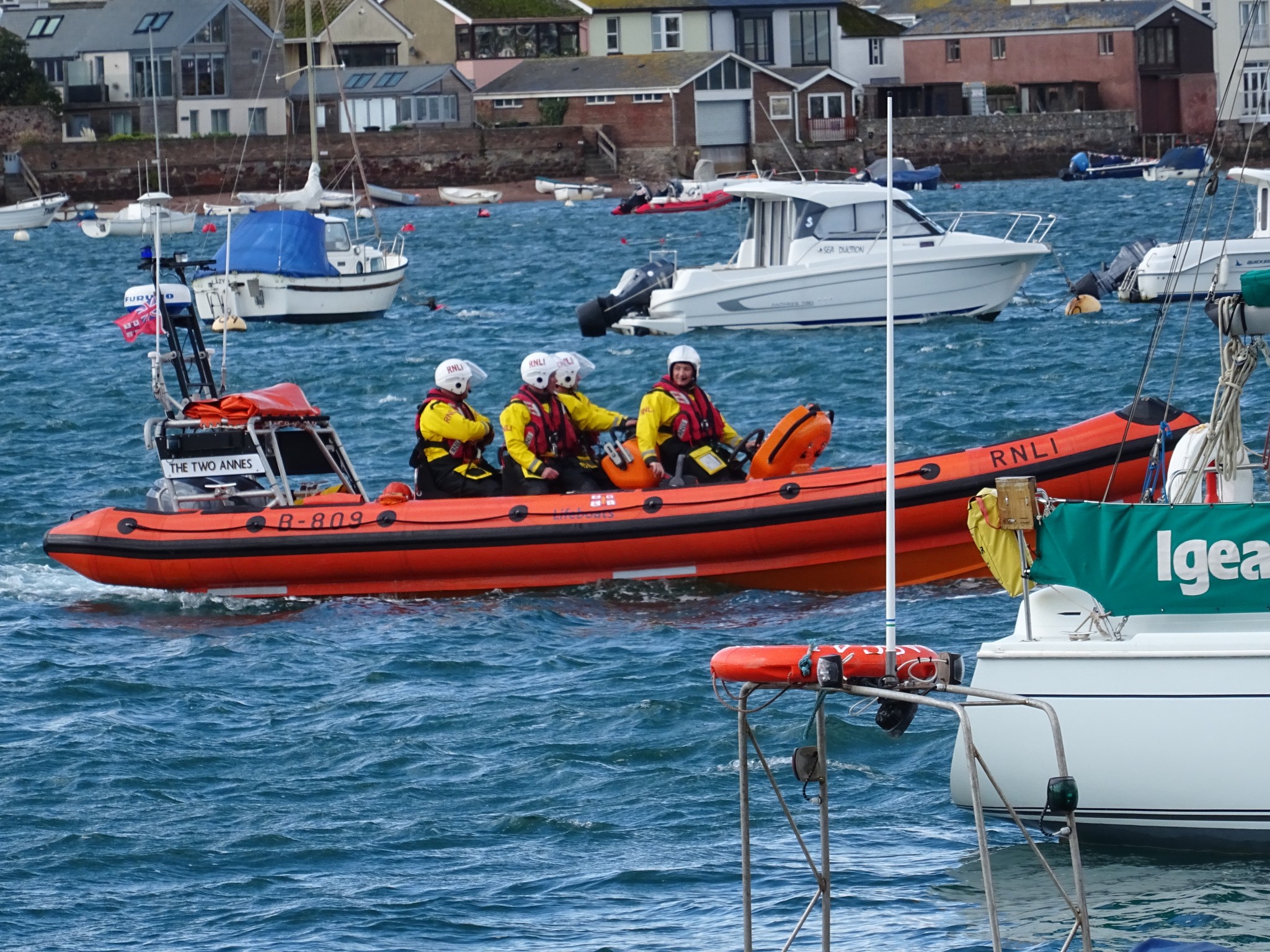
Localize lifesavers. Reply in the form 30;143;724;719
1162;423;1254;506
709;645;941;685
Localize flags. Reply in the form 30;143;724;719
401;224;415;232
113;295;167;343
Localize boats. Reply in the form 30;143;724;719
675;159;773;195
437;185;503;203
578;175;1056;336
191;0;415;325
80;201;200;237
848;158;941;190
1059;152;1158;180
611;180;734;214
0;189;71;230
55;202;100;221
45;203;1201;603
1118;0;1270;299
279;191;359;208
203;202;256;215
948;0;1270;854
365;183;421;207
1143;144;1216;181
535;177;613;201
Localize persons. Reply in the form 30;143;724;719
414;359;504;498
655;178;679;198
637;345;761;489
500;350;602;496
621;182;649;209
539;352;640;494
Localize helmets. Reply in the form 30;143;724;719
434;345;701;396
